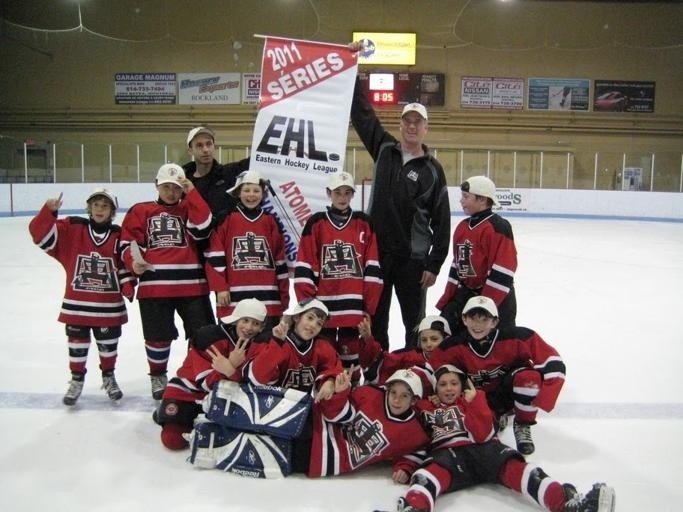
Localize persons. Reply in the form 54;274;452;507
155;296;615;512
435;176;516;330
205;169;291;339
181;127;252;265
349;41;451;351
29;187;137;407
294;171;383;389
118;164;216;406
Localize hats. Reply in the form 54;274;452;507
401;102;428;121
462;295;499;319
325;171;355;193
282;297;329;318
187;126;215;149
155;161;187;190
460;175;502;209
86;188;119;210
431;363;468;394
225;170;265;198
385;367;424;400
220;297;268;326
417;315;452;336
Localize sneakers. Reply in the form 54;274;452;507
63;368;88;407
512;417;537;456
498;413;509;431
373;496;427;512
98;364;123;401
561;481;607;512
147;369;168;401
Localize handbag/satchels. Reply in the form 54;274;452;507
180;380;314;480
